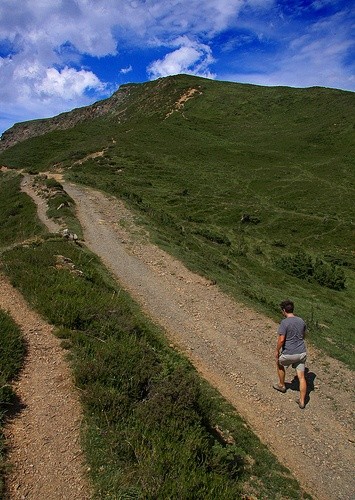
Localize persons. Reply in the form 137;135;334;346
273;300;308;409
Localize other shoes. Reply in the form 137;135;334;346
295;399;305;409
273;384;286;393
